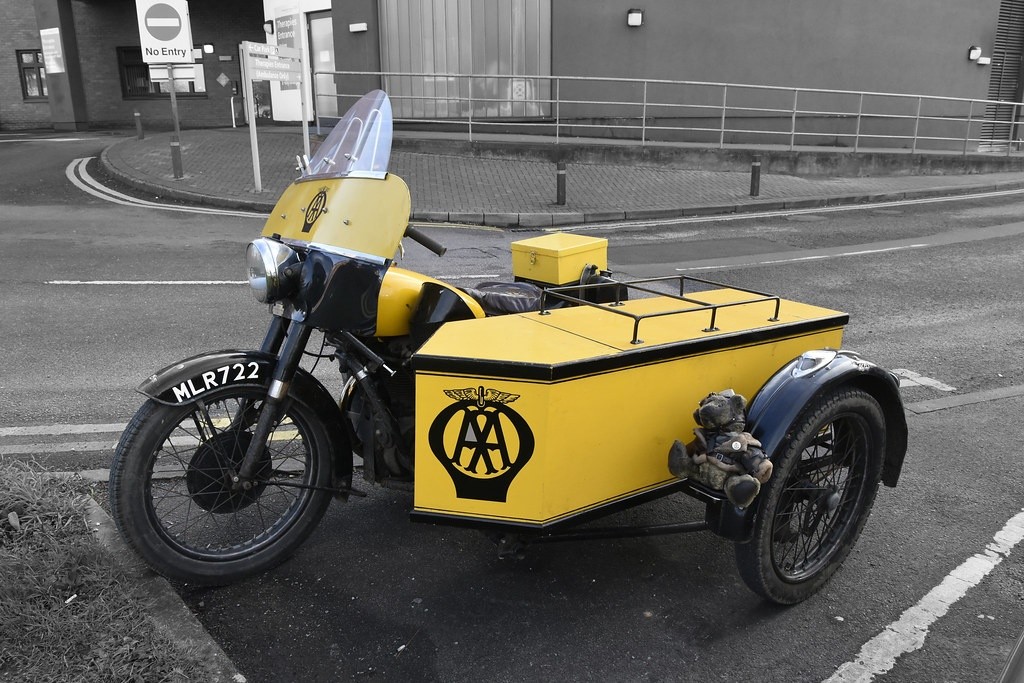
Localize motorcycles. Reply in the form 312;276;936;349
106;87;909;607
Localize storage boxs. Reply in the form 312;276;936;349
511;233;608;285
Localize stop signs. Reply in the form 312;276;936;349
135;1;194;63
